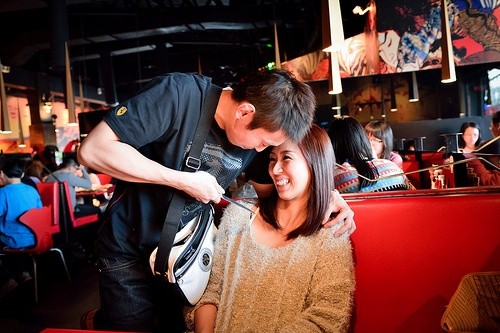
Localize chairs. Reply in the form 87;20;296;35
0;205;73;303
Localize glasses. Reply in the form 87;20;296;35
369;138;382;144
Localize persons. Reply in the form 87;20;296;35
321;117;416;193
77;70;316;333
0;141;115;297
458;111;500;187
363;120;403;170
183;123;354;333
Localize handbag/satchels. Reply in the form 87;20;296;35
149;201;218;307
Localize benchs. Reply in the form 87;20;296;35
37;150;500;333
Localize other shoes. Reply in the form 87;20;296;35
20;272;32;282
5;279;17;290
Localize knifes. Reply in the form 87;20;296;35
221;194;255;214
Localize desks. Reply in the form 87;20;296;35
75;191;106;206
